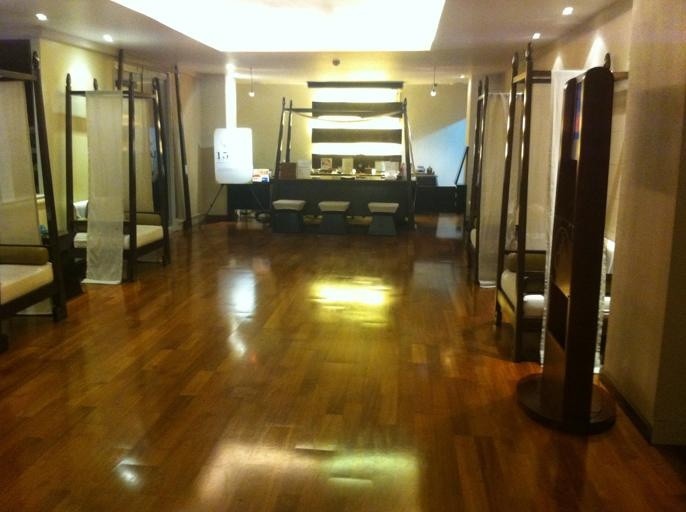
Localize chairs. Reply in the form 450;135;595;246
467;202;615;362
68;197;169;281
0;244;63;343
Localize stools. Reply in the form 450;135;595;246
368;201;399;235
273;199;305;233
318;200;351;234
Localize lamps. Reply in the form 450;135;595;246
430;65;437;96
248;68;255;97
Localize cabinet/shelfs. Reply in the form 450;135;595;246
227;181;269;221
411;176;437;212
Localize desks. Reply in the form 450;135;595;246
268;174;416;225
